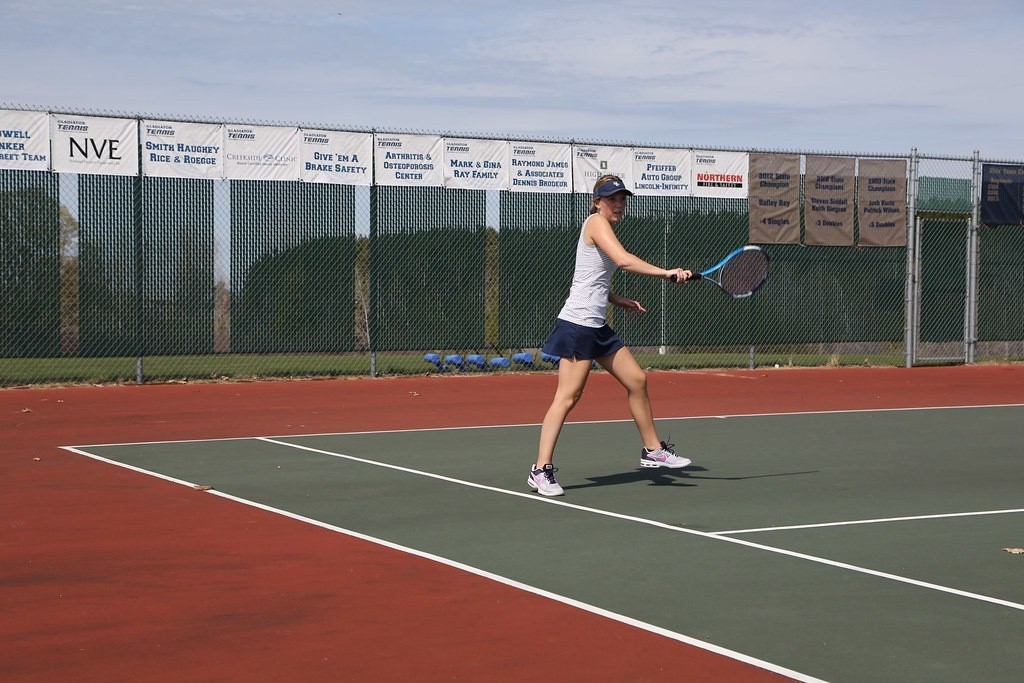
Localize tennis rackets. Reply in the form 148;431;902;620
672;245;771;300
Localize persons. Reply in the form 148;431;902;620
526;177;692;496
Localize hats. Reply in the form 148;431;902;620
592;180;633;197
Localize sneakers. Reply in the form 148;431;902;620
527;464;564;496
640;434;691;468
